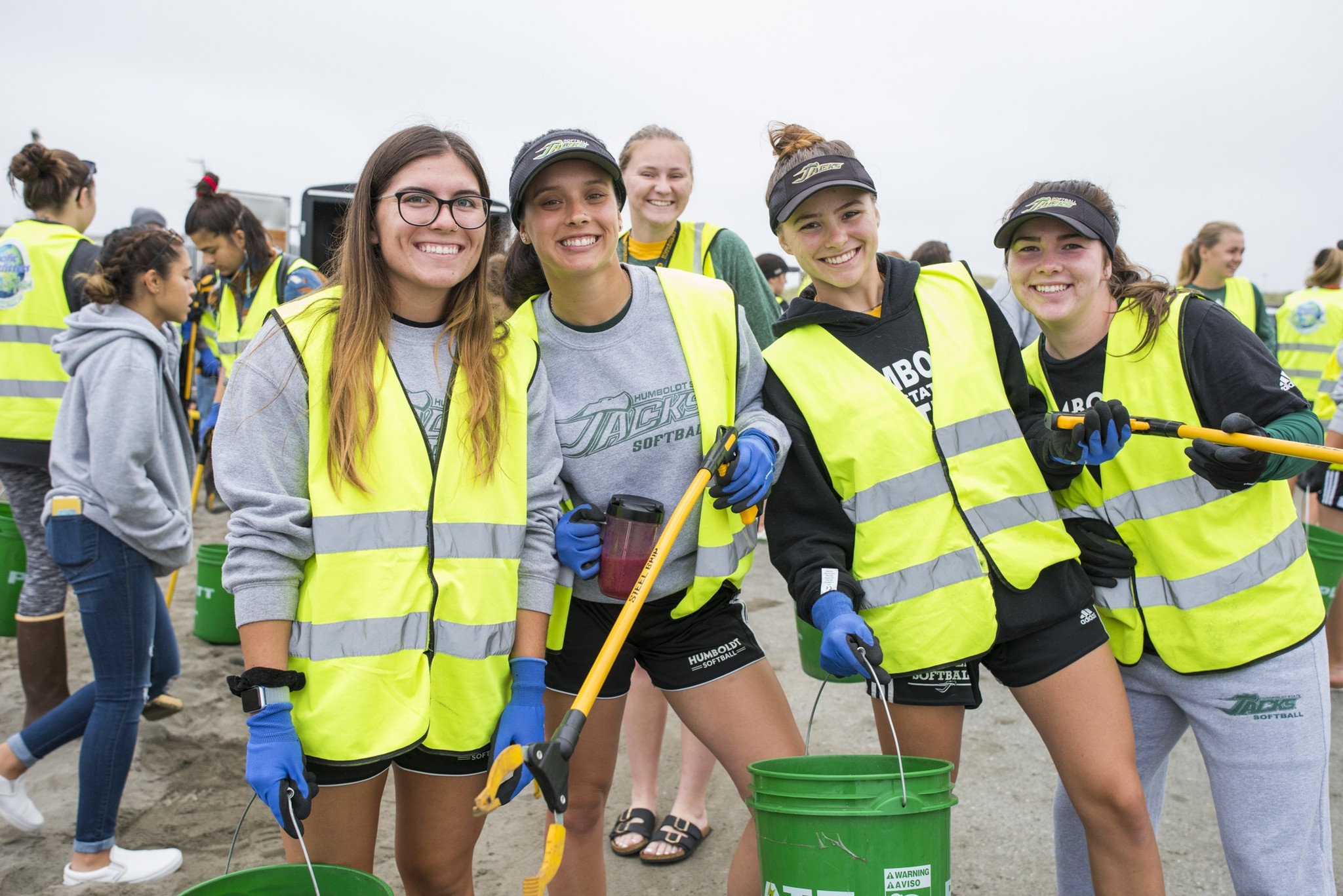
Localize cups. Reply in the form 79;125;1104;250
581;493;665;601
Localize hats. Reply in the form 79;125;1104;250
131;206;166;230
754;252;800;279
767;156;877;223
509;131;627;232
994;194;1121;261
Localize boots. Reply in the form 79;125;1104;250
15;612;72;735
141;695;184;721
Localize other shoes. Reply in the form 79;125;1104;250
63;842;182;890
0;774;45;832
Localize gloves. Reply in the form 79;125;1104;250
488;656;549;806
1296;460;1329;493
554;502;606;581
1185;412;1266;493
188;307;204;325
200;346;222;377
809;591;891;684
709;428;777;517
198;402;222;446
1051;399;1132;465
1061;517;1137;588
246;703;319;838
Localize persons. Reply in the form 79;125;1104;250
609;124;785;865
0;143;104;740
207;126;565;896
497;126;809;896
881;241;1042;353
124;168;329;514
477;250;517;330
1173;217;1280;367
750;251;790;540
0;225;197;875
1273;234;1342;687
996;178;1336;895
763;117;1174;895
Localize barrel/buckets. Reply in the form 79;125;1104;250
746;646;959;896
179;788;396;896
795;609;864;683
1301;484;1343;616
194;543;241;646
0;489;28;637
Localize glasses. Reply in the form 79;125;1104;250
76;158;97;201
373;190;492;227
146;227;185;276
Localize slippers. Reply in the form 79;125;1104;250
639;814;712;866
609;807;657;856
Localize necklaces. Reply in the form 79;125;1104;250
815;270;885;313
